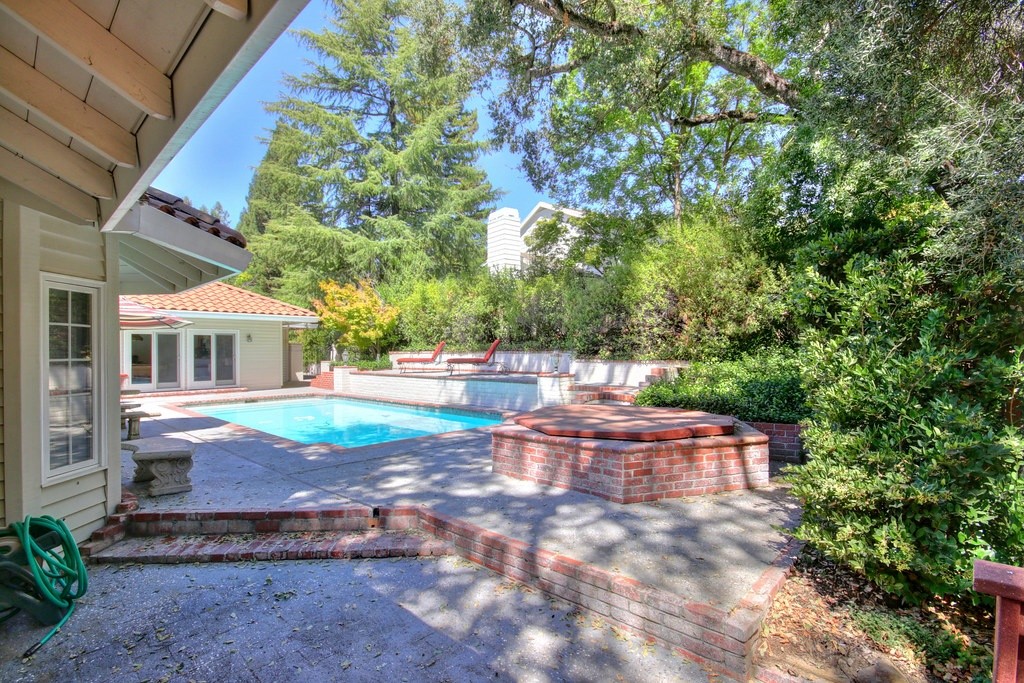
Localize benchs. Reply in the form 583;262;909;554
120;399;196;498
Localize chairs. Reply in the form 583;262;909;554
446;339;502;374
395;342;445;373
